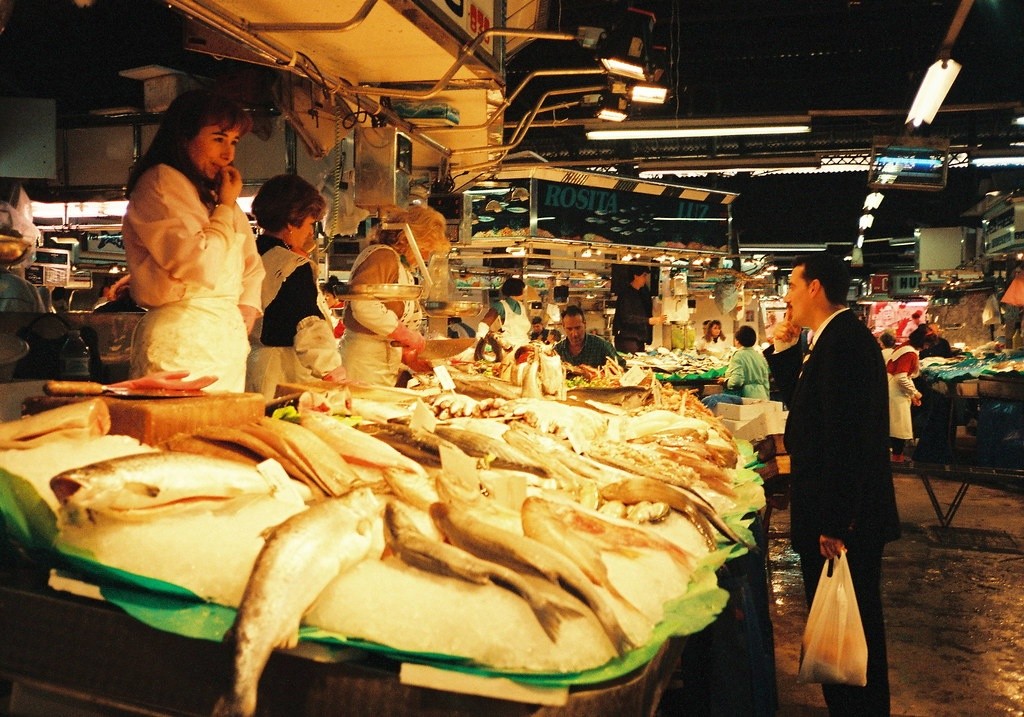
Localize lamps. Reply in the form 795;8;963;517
856;162;905;250
905;51;963;129
344;8;683;161
505;240;710;266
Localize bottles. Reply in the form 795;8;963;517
59;330;91;381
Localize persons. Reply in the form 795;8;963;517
477;278;531;351
93;274;147;312
765;314;777;343
0;222;44;311
879;333;896;359
886;324;939;461
612;265;667;354
763;252;900;717
339;204;451;388
246;174;346;399
531;317;549;342
320;276;348;337
547;329;561;344
122;89;266;393
701;320;726;345
919;324;951;360
718;325;770;400
902;313;926;337
553;305;626;379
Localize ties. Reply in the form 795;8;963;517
798;338;815;380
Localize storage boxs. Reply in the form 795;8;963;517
710;396;791;480
143;74;216;113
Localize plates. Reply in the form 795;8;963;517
419;300;482;318
470;236;525;242
322;281;424;300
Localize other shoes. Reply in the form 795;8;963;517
891;455;912;465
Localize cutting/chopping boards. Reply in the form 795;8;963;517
21;392;265;446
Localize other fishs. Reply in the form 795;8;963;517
381;502;585;644
50;452;276;517
429;502;636;659
431;397;537;418
212;485;381;717
623;347;725;375
523;495;638;613
363;420;747;543
170;412;424;496
381;467;691;565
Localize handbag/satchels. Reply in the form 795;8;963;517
796;548;868;687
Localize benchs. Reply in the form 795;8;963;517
891;462;1024;529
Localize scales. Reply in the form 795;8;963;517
320;127;434;303
529;276;698;327
419;193;484;318
